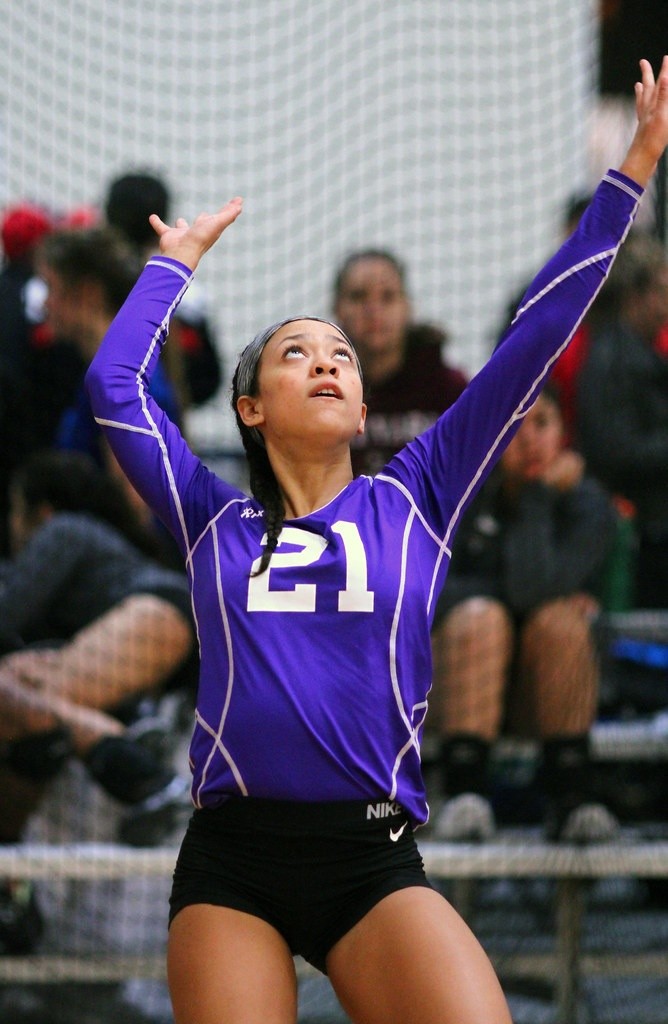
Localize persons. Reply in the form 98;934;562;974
83;50;668;1023
0;174;668;860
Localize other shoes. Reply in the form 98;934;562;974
558;784;617;840
437;787;494;840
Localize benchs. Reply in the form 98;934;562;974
0;409;668;986
